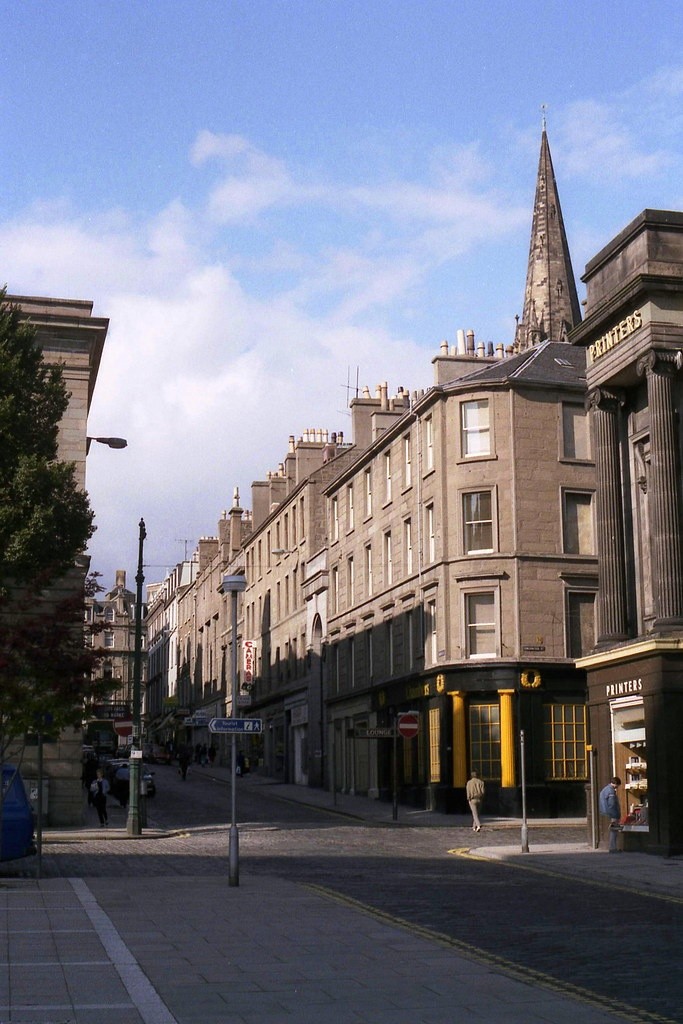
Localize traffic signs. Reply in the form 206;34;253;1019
208;717;263;734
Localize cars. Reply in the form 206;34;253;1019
148;746;170;764
109;759;156;798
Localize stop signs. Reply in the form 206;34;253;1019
114;712;135;736
397;714;419;737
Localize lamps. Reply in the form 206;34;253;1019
86;436;128;456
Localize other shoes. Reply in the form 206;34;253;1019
473;826;480;832
106;821;109;828
100;823;104;828
609;848;622;854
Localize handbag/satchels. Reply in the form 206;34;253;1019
236;766;241;774
187;766;192;775
178;767;182;776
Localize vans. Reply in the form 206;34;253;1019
0;765;38;866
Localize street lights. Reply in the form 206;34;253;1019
221;575;247;886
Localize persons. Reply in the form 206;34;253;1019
115;763;129;809
165;738;216;768
79;750;98;790
465;770;485;833
599;776;624;854
178;749;192;782
90;769;110;828
236;749;245;778
